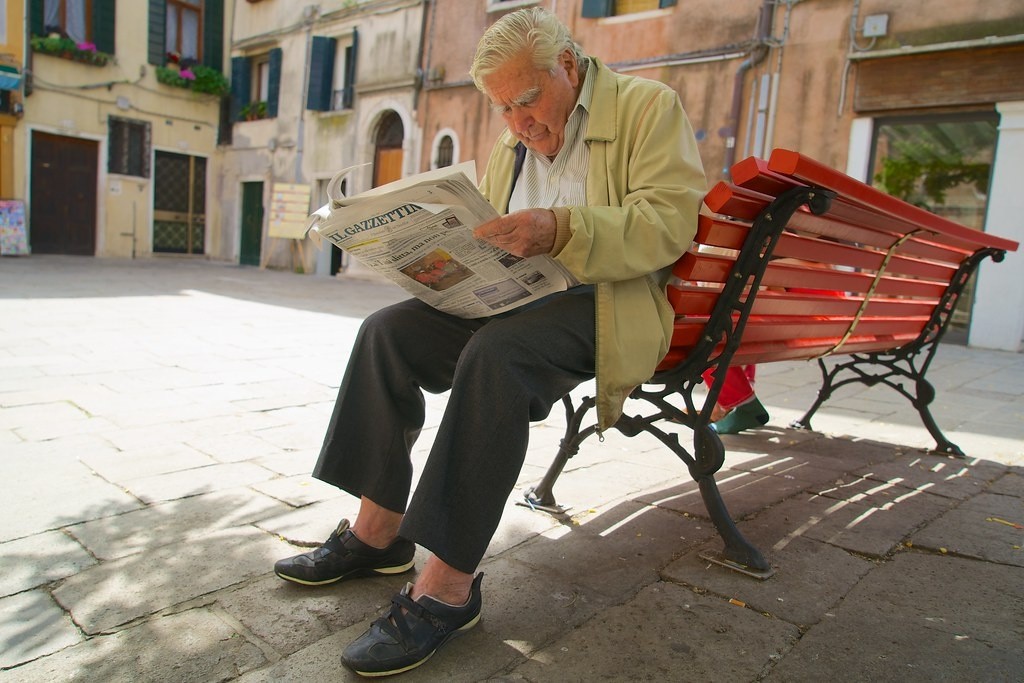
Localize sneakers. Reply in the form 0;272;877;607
341;571;485;676
274;517;414;586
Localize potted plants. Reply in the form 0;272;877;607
240;101;268;121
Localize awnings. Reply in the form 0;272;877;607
0;71;23;92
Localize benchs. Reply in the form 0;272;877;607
513;147;1020;580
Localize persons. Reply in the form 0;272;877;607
275;7;713;678
700;181;855;434
